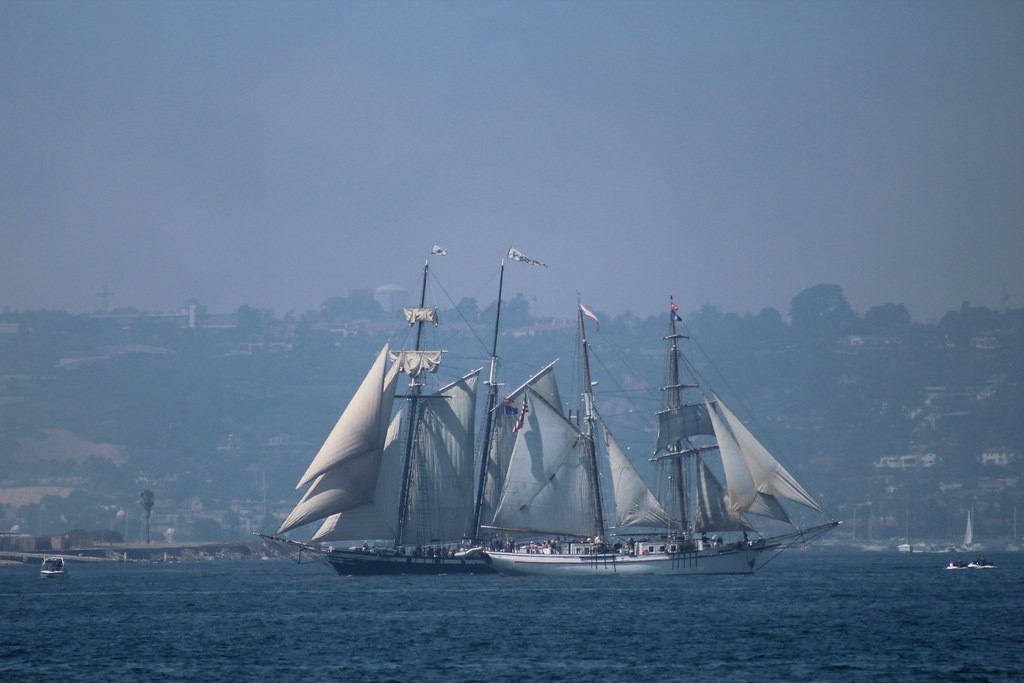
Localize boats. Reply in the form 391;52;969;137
943;561;968;570
895;544;955;555
968;561;999;569
40;556;66;577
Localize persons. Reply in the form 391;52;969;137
701;532;723;549
415;536;562;556
743;530;749;546
613;538;623;553
363;541;368;552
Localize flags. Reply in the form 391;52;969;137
507;247;548;267
432;245;447;255
513;393;529;432
503;397;518;414
671;303;682;321
578;303;600;330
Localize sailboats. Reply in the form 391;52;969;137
956;510;988;553
253;243;565;575
481;292;843;576
